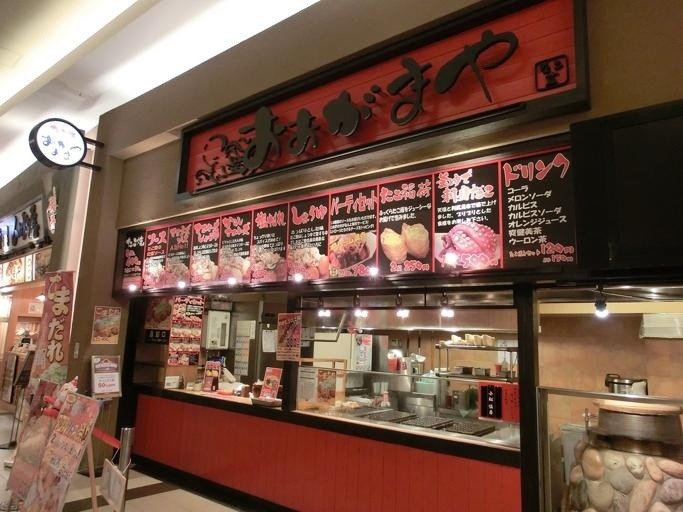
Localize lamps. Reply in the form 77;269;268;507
440;292;450;311
593;285;608;313
34;288;45;301
395;292;406;312
353;294;363;312
317;296;326;311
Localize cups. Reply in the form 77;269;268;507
496;421;517;441
373;382;380;393
327;397;353;418
381;382;388;393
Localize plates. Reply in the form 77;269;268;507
251;398;282;407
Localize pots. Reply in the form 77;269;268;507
605;373;633;394
